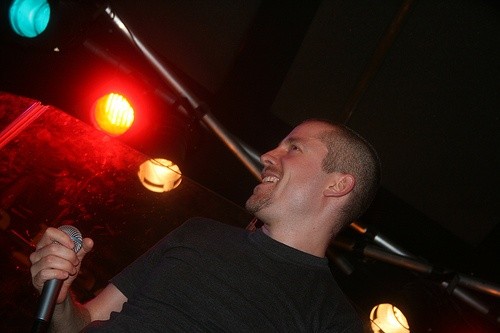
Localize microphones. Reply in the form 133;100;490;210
33;226;83;331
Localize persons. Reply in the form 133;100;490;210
29;118;383;333
